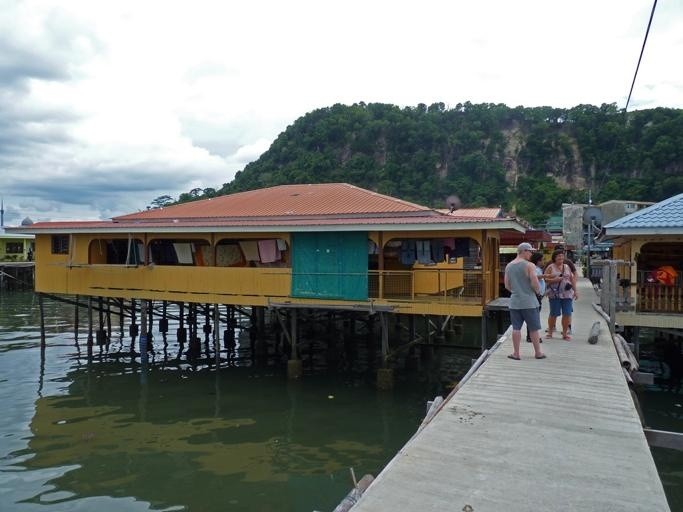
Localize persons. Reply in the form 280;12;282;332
442;246;464;299
244;260;256;267
503;242;546;359
527;252;555;343
544;249;578;340
541;244;578;335
27;247;32;261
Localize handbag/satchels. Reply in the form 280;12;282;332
548;289;559;299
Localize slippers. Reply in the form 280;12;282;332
508;354;520;360
536;353;546;358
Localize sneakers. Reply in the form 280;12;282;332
526;335;542;343
545;327;571;340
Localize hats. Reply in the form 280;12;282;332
518;242;536;251
